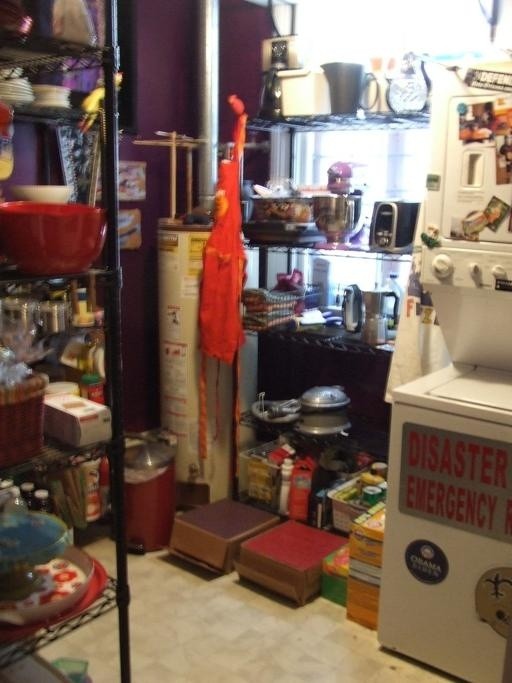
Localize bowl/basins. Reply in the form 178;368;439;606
1;200;109;276
14;183;74;204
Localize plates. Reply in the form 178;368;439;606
0;68;73;115
1;544;95;624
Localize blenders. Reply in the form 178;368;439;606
360;290;400;344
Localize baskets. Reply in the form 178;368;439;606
0;373;49;468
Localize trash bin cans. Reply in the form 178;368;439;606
124;433;176;555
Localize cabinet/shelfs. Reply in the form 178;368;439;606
229;113;432;498
0;0;132;683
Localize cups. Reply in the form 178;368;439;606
321;61;380;117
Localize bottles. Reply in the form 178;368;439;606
384;273;404;339
278;457;295;514
1;478;55;516
359;468;384;494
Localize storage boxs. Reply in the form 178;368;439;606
236;440;374;528
344;559;382;629
231;521;346;607
318;544;349;604
157;501;279;579
348;500;385;570
278;69;333;118
45;394;113;449
